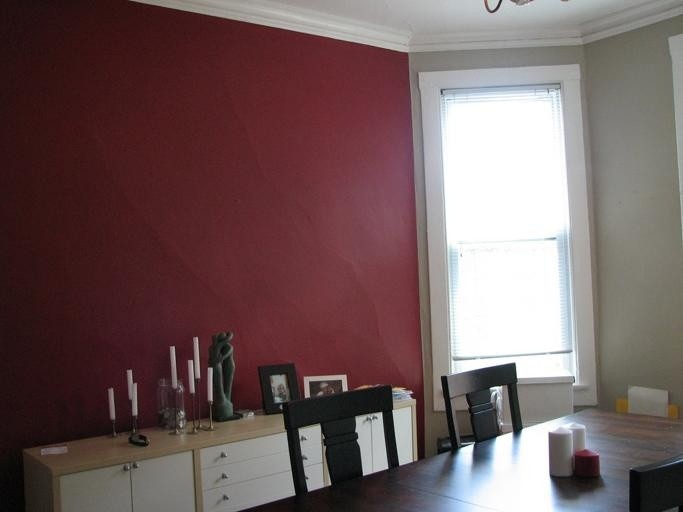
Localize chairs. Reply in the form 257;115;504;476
629;454;683;512
439;361;523;450
282;384;399;496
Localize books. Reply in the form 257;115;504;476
355;384;413;402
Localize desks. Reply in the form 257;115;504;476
238;406;683;512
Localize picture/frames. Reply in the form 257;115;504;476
304;374;349;399
258;362;299;415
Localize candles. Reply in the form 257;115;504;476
548;426;573;478
169;345;178;390
187;359;195;394
573;448;600;478
107;387;116;421
193;336;200;379
207;367;214;403
131;382;139;417
125;369;134;401
561;422;586;472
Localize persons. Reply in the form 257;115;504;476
315;381;337;397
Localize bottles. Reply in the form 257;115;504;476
155;375;187;432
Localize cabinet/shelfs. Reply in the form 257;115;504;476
197;407;328;512
24;425;199;512
327;397;418;486
